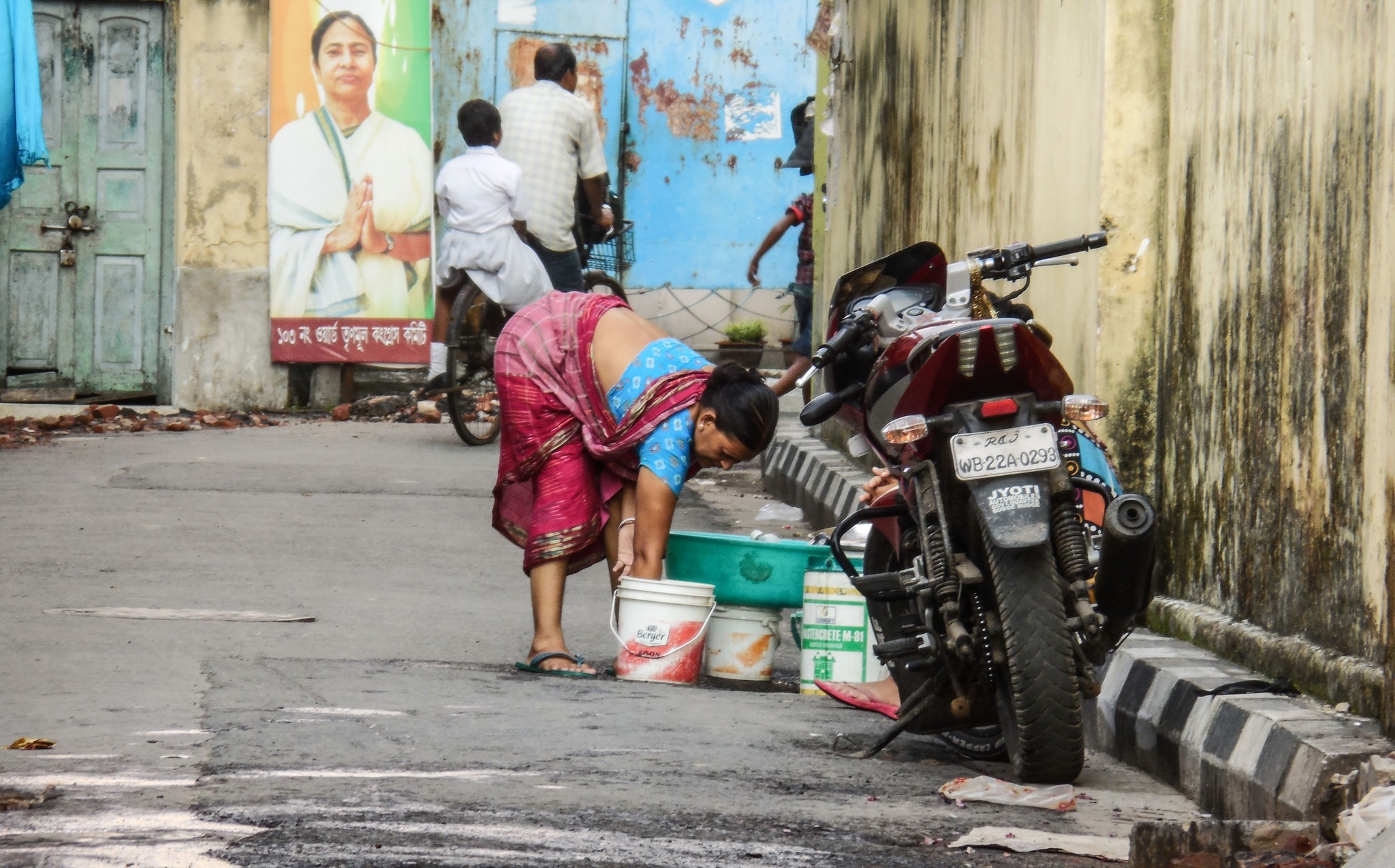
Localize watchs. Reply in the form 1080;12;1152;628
381;231;395;254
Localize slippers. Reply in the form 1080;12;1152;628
813;677;900;721
514;652;598;679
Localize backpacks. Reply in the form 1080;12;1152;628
578;190;625;241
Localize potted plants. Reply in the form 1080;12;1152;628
779;304;796;368
715;320;770;367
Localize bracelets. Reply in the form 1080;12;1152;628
618;517;635;529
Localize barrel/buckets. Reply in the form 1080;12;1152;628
798;556;890;696
609;576;716;685
705;604;784;681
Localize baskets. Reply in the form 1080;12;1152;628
582;219;636;271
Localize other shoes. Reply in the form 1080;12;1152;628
417;372;449;399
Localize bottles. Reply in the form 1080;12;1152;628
750;529;780;542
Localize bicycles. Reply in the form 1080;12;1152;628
419;192;637;447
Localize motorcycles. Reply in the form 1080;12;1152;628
792;233;1162;789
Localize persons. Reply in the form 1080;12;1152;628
814;415;1117;716
495;42;614;293
748;192;812;405
487;296;780;681
268;11;430;320
418;99;554;400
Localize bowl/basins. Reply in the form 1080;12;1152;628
808;523;872;556
666;530;832;608
